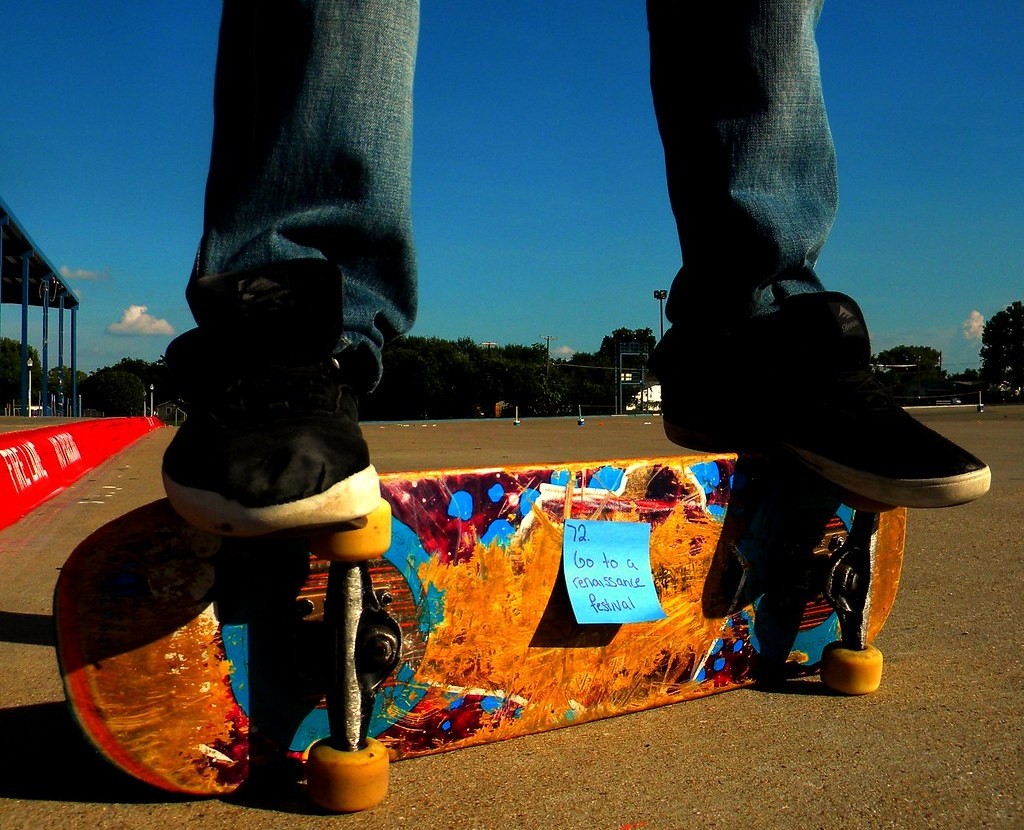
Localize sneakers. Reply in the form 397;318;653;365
660;289;993;508
161;258;379;537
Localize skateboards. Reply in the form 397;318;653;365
48;448;912;812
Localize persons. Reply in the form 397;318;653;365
161;1;993;538
